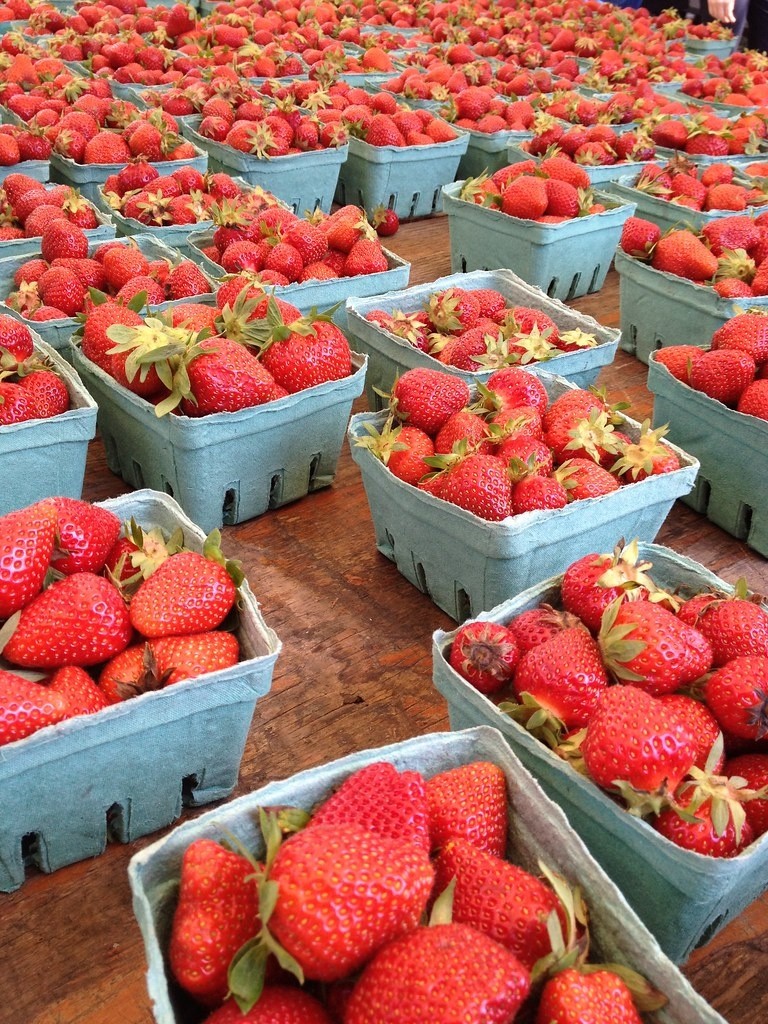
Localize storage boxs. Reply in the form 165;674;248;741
0;0;768;1024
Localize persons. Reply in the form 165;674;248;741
604;0;768;55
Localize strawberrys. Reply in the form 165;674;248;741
0;0;768;1024
